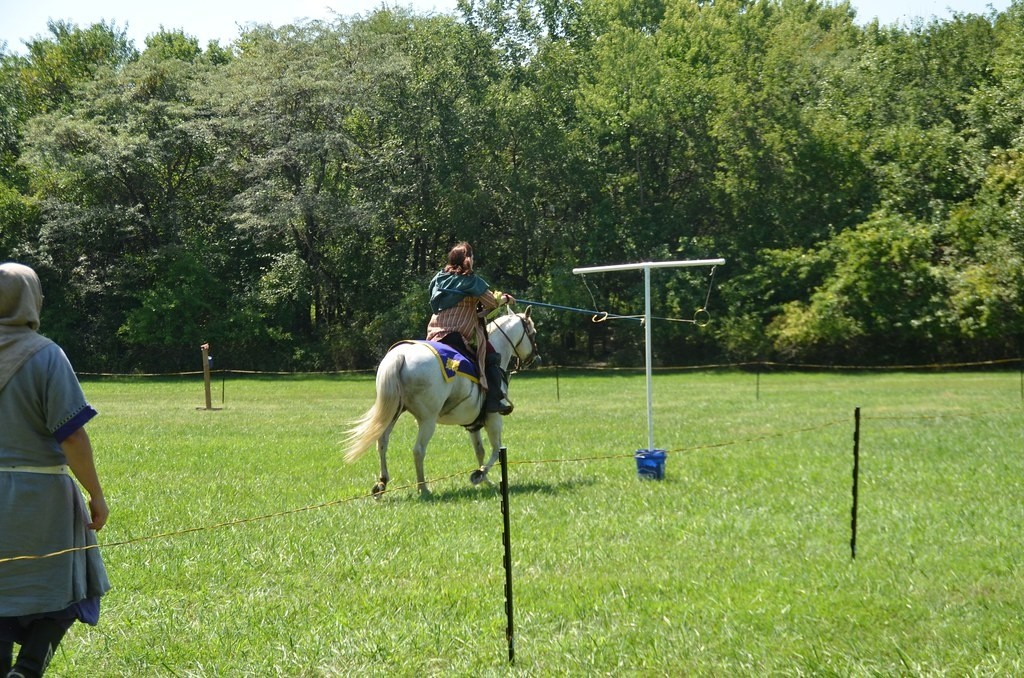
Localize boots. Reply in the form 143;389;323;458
485;352;513;415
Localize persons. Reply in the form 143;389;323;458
0;261;108;678
426;243;516;414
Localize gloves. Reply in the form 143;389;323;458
504;294;516;305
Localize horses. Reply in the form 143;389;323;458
337;304;543;501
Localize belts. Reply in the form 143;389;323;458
0;464;68;474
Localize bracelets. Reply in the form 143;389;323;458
503;293;510;303
